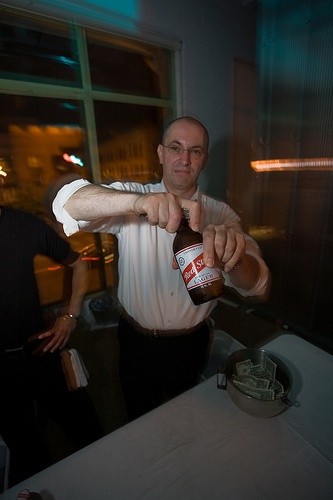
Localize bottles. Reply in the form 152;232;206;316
172;207;225;306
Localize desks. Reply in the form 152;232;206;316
1;334;333;500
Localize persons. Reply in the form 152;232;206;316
0;205;104;490
51;117;272;423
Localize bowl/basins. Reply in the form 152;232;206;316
216;347;293;419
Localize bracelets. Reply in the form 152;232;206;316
63;311;78;320
215;259;240;273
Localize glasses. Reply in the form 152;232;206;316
160;143;207;160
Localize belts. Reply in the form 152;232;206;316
121;307;209;337
